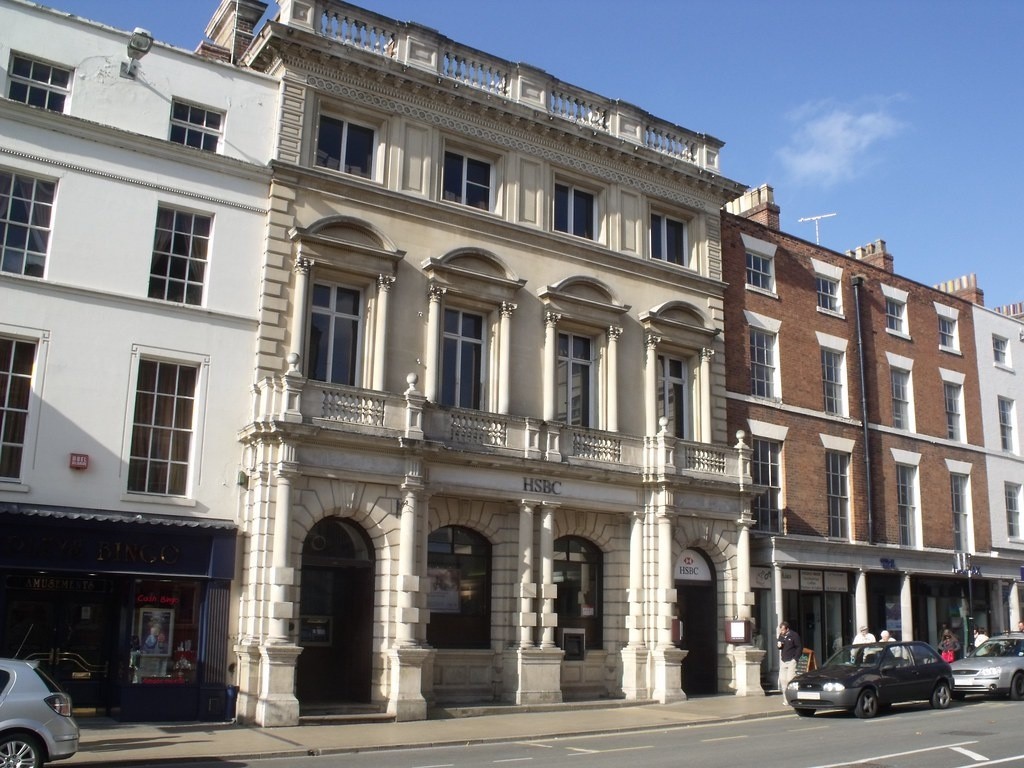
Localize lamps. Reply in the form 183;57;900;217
119;27;154;80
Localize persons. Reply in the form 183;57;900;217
851;625;876;660
1019;621;1024;632
1005;644;1015;655
938;623;961;662
878;629;900;651
989;643;1000;656
974;624;991;657
776;622;803;706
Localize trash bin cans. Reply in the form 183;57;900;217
197;682;227;722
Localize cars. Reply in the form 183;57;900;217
0;658;80;768
949;632;1024;702
785;640;955;719
23;651;107;692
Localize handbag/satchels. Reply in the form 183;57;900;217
942;650;954;662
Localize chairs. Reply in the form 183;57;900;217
865;654;906;666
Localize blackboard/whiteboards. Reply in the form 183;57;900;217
795;650;818;677
